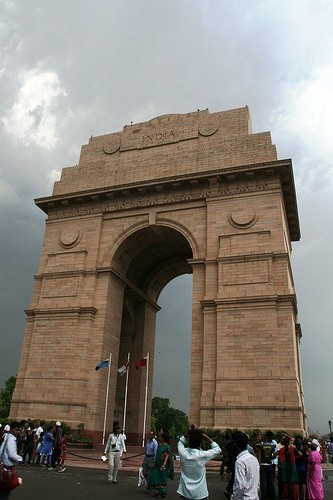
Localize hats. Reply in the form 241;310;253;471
56;421;62;426
250;429;261;437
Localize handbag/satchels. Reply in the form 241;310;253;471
1;462;18;483
138;466;148;487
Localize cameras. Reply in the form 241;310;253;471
112;444;116;448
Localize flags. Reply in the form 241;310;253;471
135;359;147;369
118;362;130;373
96;358;110;370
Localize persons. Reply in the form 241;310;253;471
0;417;43;466
224;429;324;500
323;436;333;464
0;421;23;500
177;423;222;500
138;431;174;498
104;426;127;482
40;421;67;472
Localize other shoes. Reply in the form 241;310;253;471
19;462;67;472
152;492;167;496
224;491;231;500
108;481;117;484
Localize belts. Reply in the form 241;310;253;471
114;450;121;452
146;454;154;457
